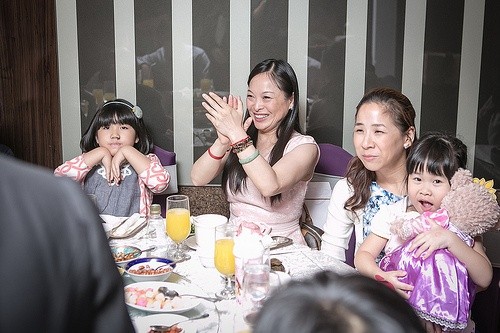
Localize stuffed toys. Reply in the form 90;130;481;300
379;168;500;333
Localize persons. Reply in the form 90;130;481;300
191;58;321;246
53;99;172;220
252;271;427;333
319;89;442;333
354;131;492;300
0;163;136;333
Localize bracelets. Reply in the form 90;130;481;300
239;148;259;164
231;134;253;153
208;148;226;159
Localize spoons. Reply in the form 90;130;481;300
150;313;209;333
158;287;224;302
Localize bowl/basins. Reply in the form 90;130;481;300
124;257;176;282
102;223;112;241
111;244;142;268
116;265;125;277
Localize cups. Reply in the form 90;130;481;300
88;193;97;207
143;70;154;88
200;73;213;92
192;214;228;269
240;270;283;330
93;81;115;105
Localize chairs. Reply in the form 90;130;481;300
148;145;176;217
299;143;355;268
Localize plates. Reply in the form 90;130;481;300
185;236;197;250
99;214;122;229
131;314;197;333
123;281;204;313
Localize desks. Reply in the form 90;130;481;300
108;218;355;333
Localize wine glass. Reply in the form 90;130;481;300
214;224;271;323
165;195;192;262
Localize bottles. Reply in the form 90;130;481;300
146;203;166;258
